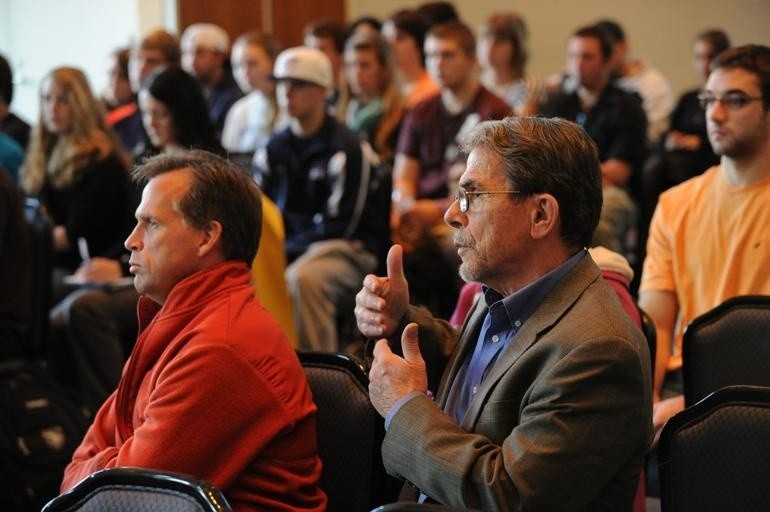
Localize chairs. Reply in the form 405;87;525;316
683;294;770;409
37;466;232;512
658;386;769;512
295;349;401;512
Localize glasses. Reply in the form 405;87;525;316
452;186;521;211
696;91;763;108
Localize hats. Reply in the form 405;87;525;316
181;24;229;52
263;45;334;89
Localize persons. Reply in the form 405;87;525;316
0;0;769;451
55;143;334;512
350;111;658;512
